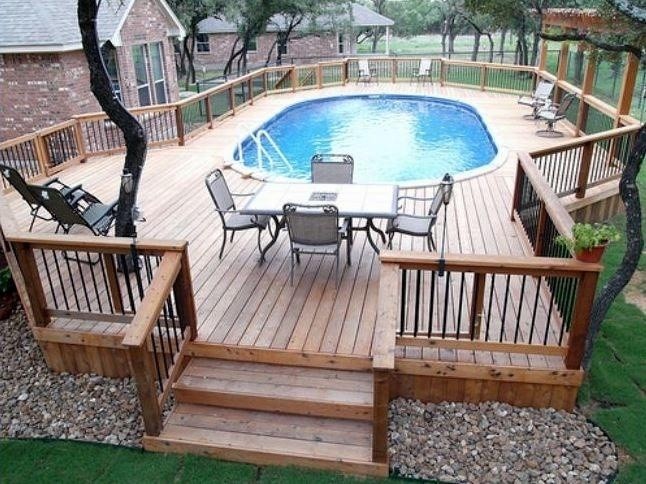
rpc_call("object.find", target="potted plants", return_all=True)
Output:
[0,267,14,320]
[549,220,622,263]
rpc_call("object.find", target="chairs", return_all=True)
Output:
[0,163,146,266]
[518,79,578,137]
[412,60,435,87]
[353,58,378,87]
[204,152,454,290]
